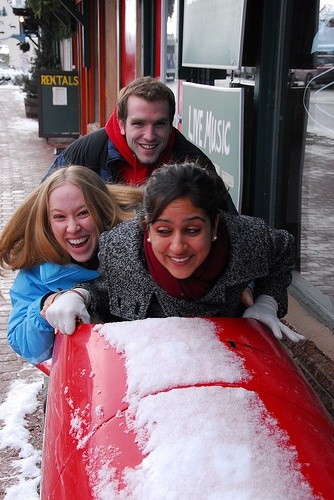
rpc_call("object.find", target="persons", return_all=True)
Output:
[0,165,143,366]
[41,74,254,309]
[43,162,307,344]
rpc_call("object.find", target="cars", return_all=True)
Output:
[290,49,334,89]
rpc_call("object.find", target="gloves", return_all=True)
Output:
[45,288,90,336]
[241,295,304,343]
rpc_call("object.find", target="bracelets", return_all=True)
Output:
[51,290,87,305]
